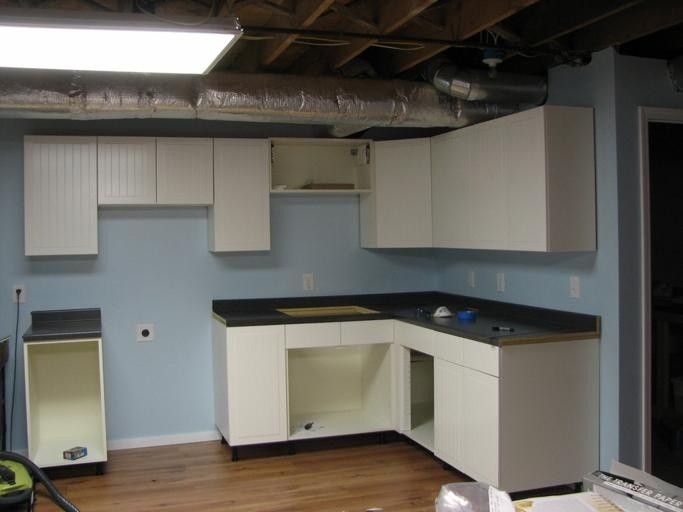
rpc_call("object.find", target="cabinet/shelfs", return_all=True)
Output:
[359,137,432,249]
[432,105,596,252]
[267,137,374,196]
[98,136,156,205]
[24,135,98,256]
[156,137,213,207]
[22,308,108,482]
[208,138,270,253]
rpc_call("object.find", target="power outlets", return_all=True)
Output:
[12,284,25,304]
[136,323,154,343]
